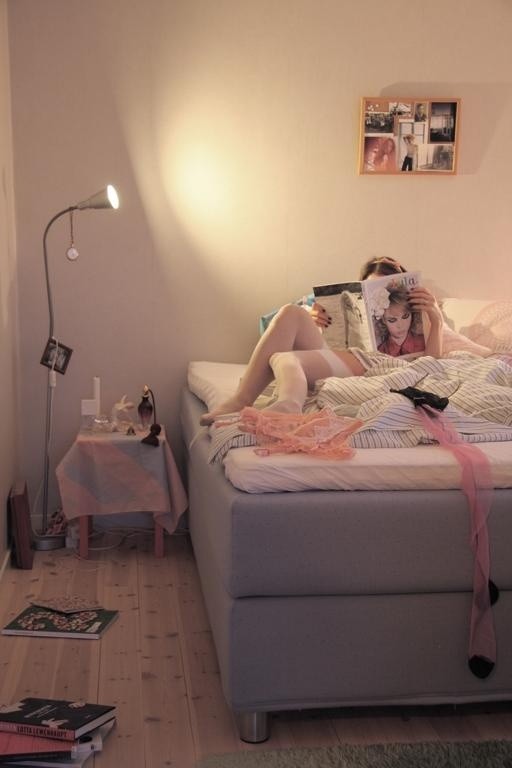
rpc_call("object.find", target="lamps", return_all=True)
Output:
[137,384,163,449]
[30,182,127,552]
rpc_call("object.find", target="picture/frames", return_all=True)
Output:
[355,94,465,177]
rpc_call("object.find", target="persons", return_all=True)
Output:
[377,139,395,171]
[198,256,444,427]
[402,134,419,172]
[415,104,426,122]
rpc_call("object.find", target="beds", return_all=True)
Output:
[175,359,512,746]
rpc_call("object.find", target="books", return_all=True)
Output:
[306,271,427,360]
[2,605,119,640]
[0,696,119,768]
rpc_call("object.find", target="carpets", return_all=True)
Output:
[196,737,512,767]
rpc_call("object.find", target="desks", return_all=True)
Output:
[54,421,190,561]
